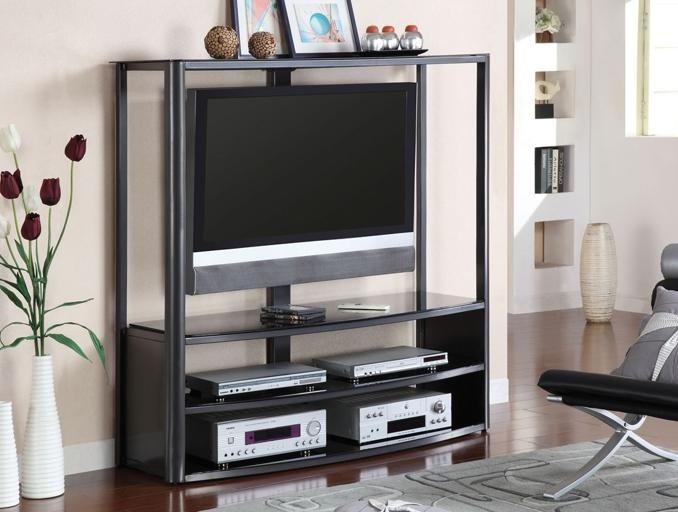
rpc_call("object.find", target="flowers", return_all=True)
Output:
[0,126,111,378]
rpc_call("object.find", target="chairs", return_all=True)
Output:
[534,242,678,498]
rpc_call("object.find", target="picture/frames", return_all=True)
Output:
[230,1,361,61]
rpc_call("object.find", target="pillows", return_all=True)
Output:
[607,285,678,387]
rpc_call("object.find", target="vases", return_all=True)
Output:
[0,355,65,509]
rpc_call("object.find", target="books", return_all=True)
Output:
[535,147,567,194]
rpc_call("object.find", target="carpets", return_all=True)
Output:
[196,437,676,511]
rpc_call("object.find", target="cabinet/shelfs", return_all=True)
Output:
[515,0,591,313]
[115,54,490,485]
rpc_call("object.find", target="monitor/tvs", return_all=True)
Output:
[185,82,418,295]
